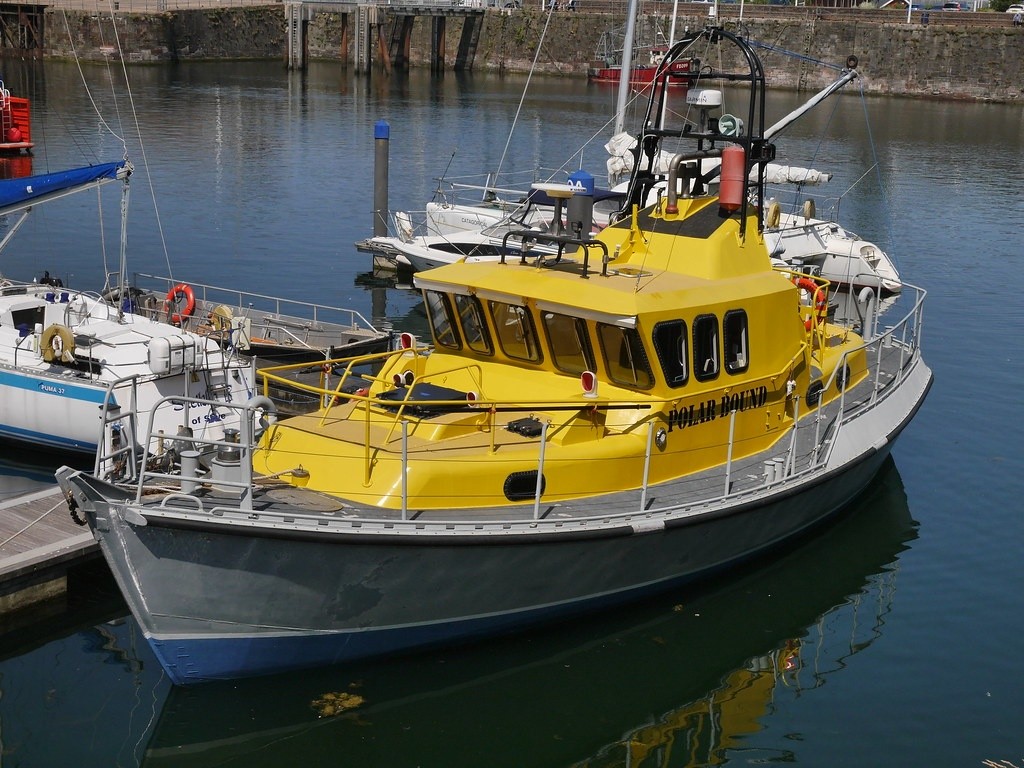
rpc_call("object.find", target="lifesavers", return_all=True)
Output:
[791,276,827,332]
[40,324,76,365]
[766,202,781,229]
[164,283,195,323]
[803,198,818,221]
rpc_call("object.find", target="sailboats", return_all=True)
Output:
[354,1,908,293]
[130,454,924,767]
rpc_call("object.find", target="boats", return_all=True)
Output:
[52,202,932,690]
[0,279,215,464]
[584,51,697,88]
[89,282,396,362]
